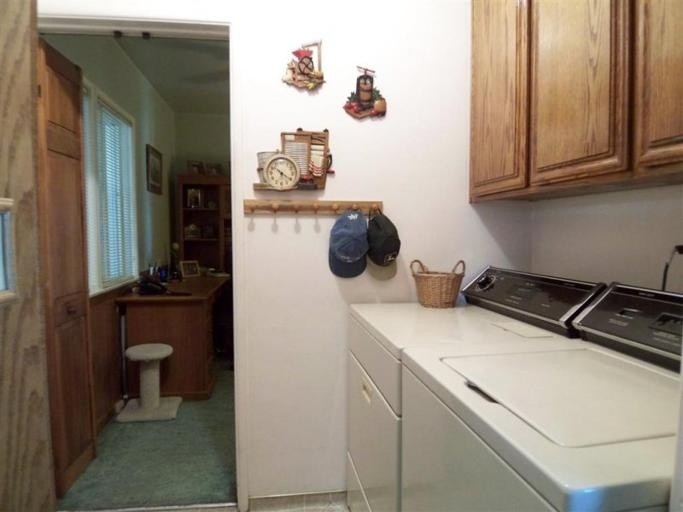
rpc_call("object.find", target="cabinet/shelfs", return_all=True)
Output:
[177,173,232,274]
[634,0,683,192]
[465,0,529,207]
[528,0,634,202]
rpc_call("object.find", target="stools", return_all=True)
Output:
[114,342,184,423]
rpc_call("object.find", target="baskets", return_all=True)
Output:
[410,259,465,309]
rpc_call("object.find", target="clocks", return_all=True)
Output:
[262,152,301,191]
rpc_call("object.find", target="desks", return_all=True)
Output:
[116,276,230,402]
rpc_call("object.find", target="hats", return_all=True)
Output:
[329,208,369,278]
[367,207,400,266]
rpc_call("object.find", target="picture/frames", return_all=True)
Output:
[146,141,164,196]
[178,258,200,277]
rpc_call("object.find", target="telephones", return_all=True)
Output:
[137,280,166,296]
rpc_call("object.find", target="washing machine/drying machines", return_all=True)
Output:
[402,280,683,512]
